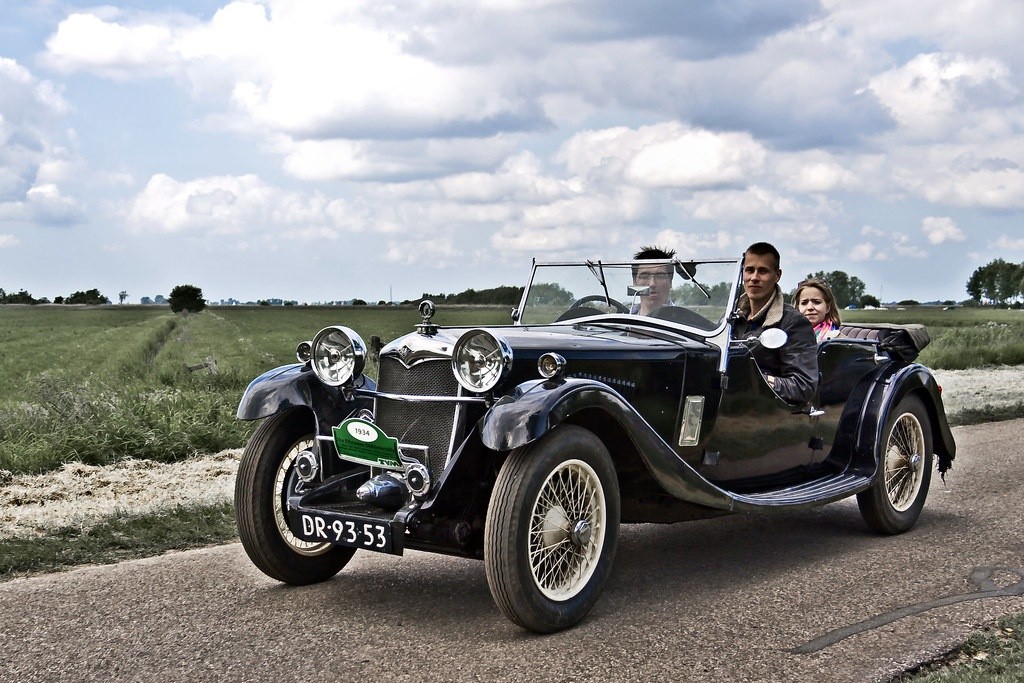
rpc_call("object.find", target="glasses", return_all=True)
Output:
[798,279,827,288]
[636,274,670,282]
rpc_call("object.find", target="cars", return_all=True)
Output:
[233,256,955,636]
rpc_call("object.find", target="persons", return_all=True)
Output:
[791,279,846,344]
[628,246,675,315]
[720,242,818,405]
